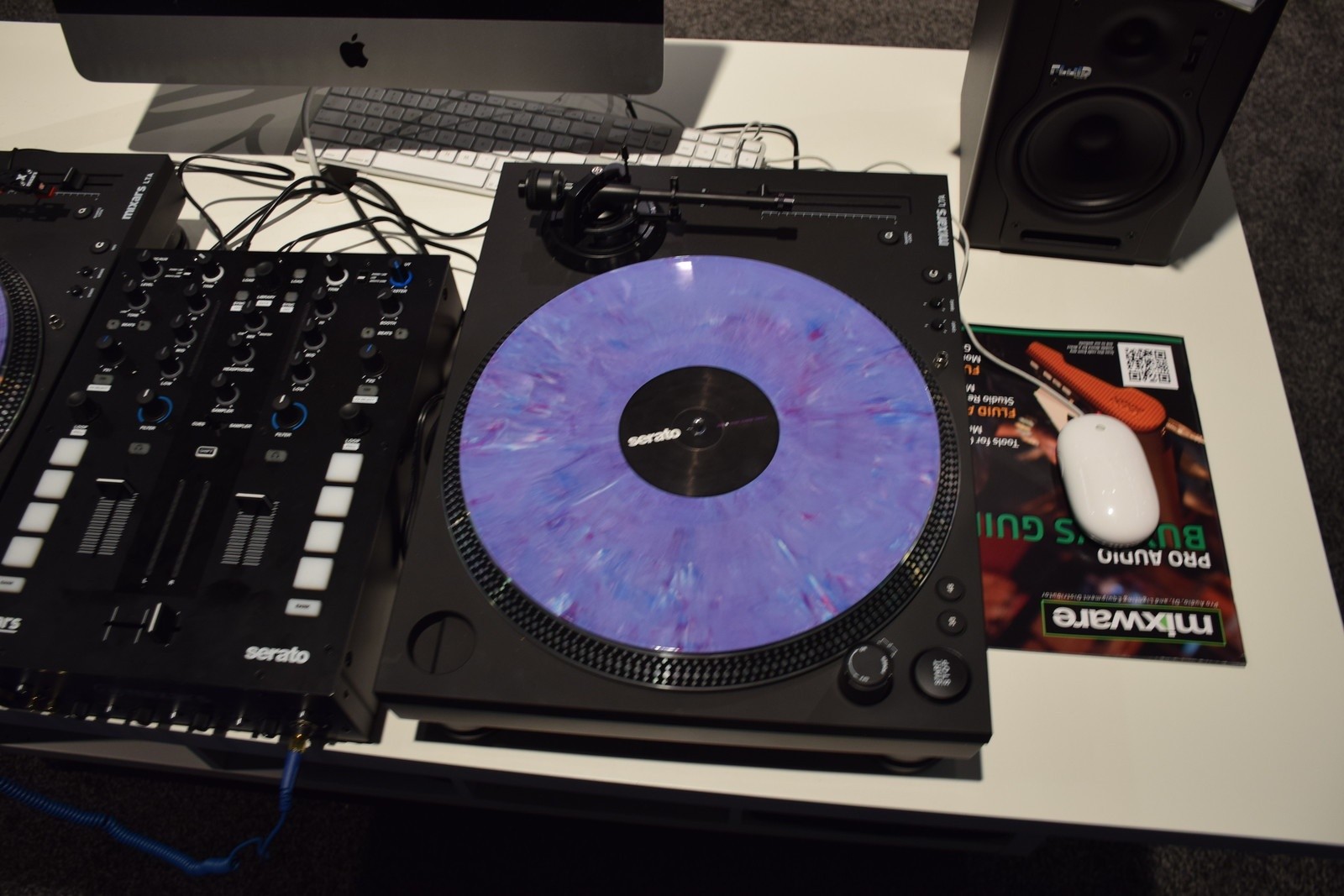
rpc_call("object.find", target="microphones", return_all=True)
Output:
[517,164,795,213]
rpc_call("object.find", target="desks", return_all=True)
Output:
[2,19,1344,895]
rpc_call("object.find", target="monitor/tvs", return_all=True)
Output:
[49,0,665,96]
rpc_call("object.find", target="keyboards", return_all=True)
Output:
[288,90,766,199]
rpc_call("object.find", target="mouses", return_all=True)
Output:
[1054,411,1162,548]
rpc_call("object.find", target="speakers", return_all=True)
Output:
[956,0,1290,266]
